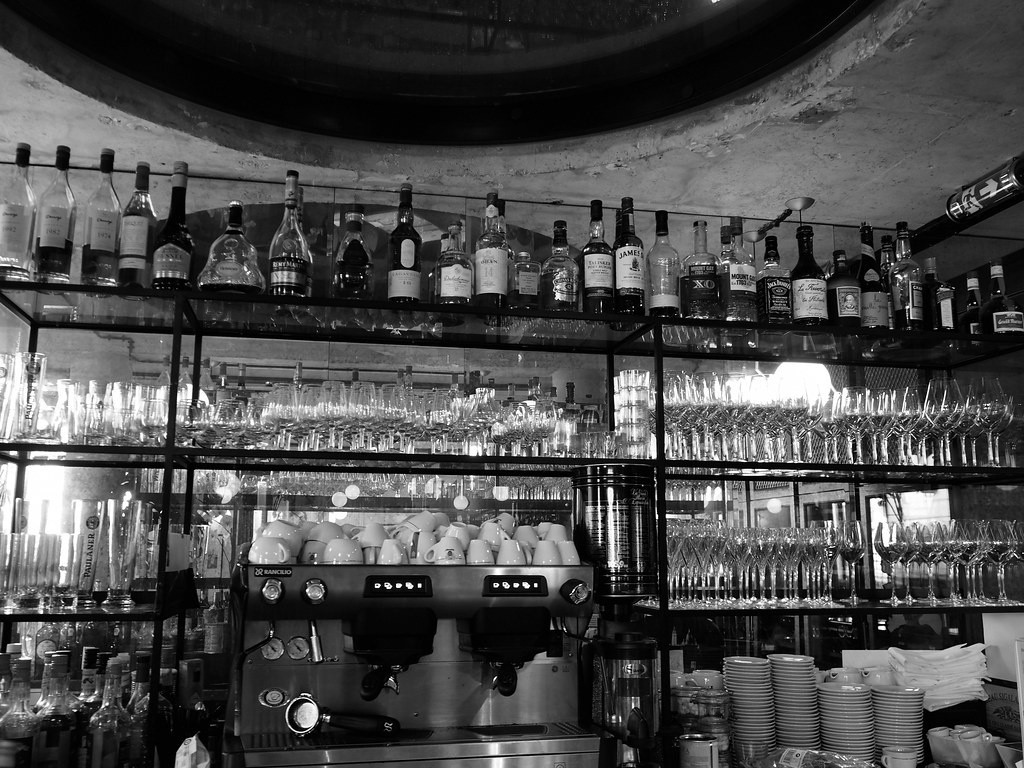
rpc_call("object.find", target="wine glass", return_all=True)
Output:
[875,519,1024,608]
[636,518,868,609]
[139,380,559,458]
[650,370,1024,468]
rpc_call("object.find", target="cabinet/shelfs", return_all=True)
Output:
[0,280,1024,768]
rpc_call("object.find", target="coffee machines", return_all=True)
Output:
[579,628,664,768]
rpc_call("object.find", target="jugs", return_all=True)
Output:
[671,688,733,768]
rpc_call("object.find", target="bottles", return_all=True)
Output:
[0,142,1023,356]
[0,644,180,768]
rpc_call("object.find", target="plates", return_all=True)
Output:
[722,654,821,767]
[815,682,926,765]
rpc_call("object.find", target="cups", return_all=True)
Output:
[927,724,1001,767]
[1,349,191,447]
[881,747,917,768]
[668,669,725,693]
[816,666,895,685]
[246,510,580,566]
[556,368,653,461]
[1,496,211,612]
